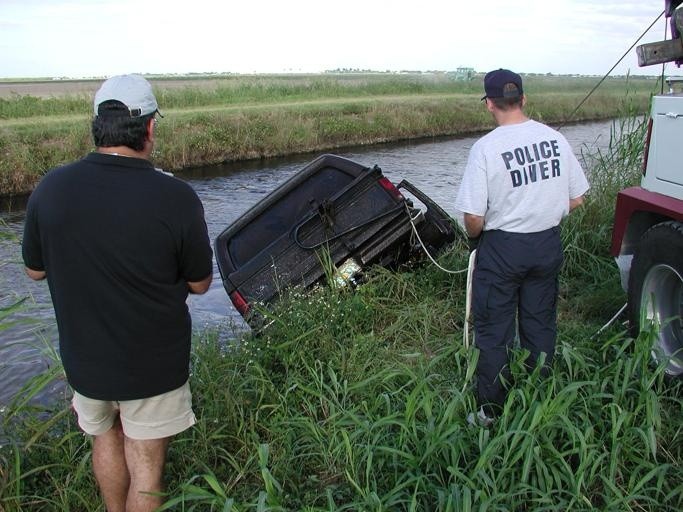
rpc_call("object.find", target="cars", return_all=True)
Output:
[212,154,468,341]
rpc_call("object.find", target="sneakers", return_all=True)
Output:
[466,406,502,429]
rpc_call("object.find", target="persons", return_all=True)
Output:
[22,74,213,512]
[454,68,590,427]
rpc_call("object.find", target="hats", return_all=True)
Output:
[93,73,165,123]
[479,68,524,103]
